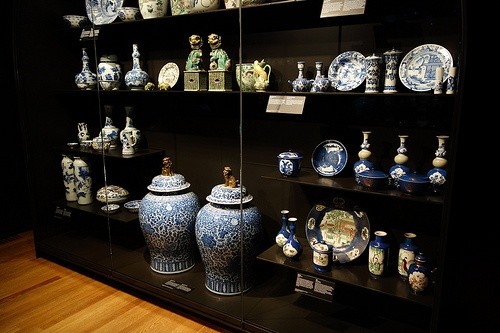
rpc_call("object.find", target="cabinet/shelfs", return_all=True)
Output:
[22,0,500,333]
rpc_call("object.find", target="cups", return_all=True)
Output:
[235,64,255,90]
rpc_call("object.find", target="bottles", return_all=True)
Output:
[62,105,262,296]
[291,61,329,93]
[276,209,334,272]
[74,44,149,90]
[354,130,450,294]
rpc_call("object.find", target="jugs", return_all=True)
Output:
[252,60,271,91]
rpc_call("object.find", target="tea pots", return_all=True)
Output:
[77,123,90,146]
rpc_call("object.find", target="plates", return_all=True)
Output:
[305,197,370,264]
[158,62,179,88]
[328,51,367,92]
[85,0,123,26]
[399,43,453,92]
[310,140,348,177]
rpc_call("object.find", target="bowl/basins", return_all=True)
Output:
[63,15,87,27]
[101,204,120,213]
[357,168,389,190]
[91,136,111,152]
[277,150,303,179]
[67,143,78,149]
[124,200,141,212]
[398,170,433,195]
[116,7,139,22]
